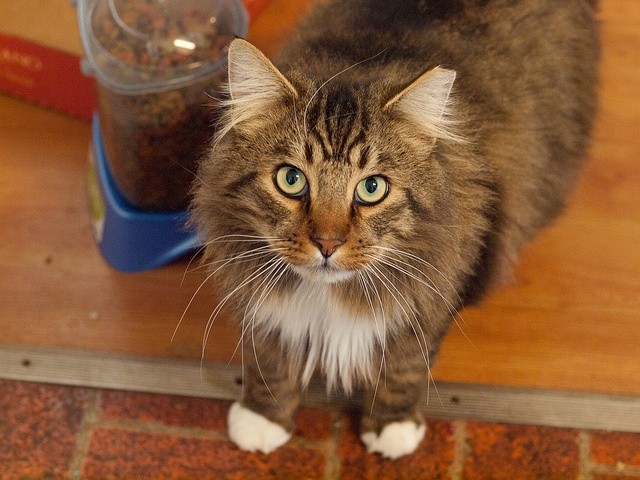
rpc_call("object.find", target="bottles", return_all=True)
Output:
[73,0,246,214]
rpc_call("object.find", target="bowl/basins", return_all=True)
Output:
[88,113,212,272]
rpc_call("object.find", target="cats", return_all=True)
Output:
[172,0,601,461]
